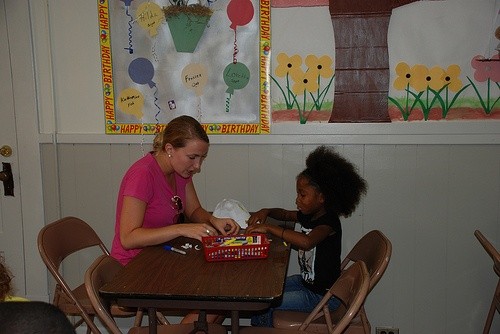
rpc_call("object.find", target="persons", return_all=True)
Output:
[245,145,369,328]
[0,255,30,302]
[109,115,240,276]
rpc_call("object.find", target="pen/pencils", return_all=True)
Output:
[163,245,187,255]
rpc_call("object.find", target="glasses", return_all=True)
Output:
[170,196,185,224]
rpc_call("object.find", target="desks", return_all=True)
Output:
[99,212,296,334]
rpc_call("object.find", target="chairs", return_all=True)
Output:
[37,216,392,334]
[474,230,500,334]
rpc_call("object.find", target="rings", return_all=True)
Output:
[206,229,210,234]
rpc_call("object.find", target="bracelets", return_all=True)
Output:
[282,229,285,240]
